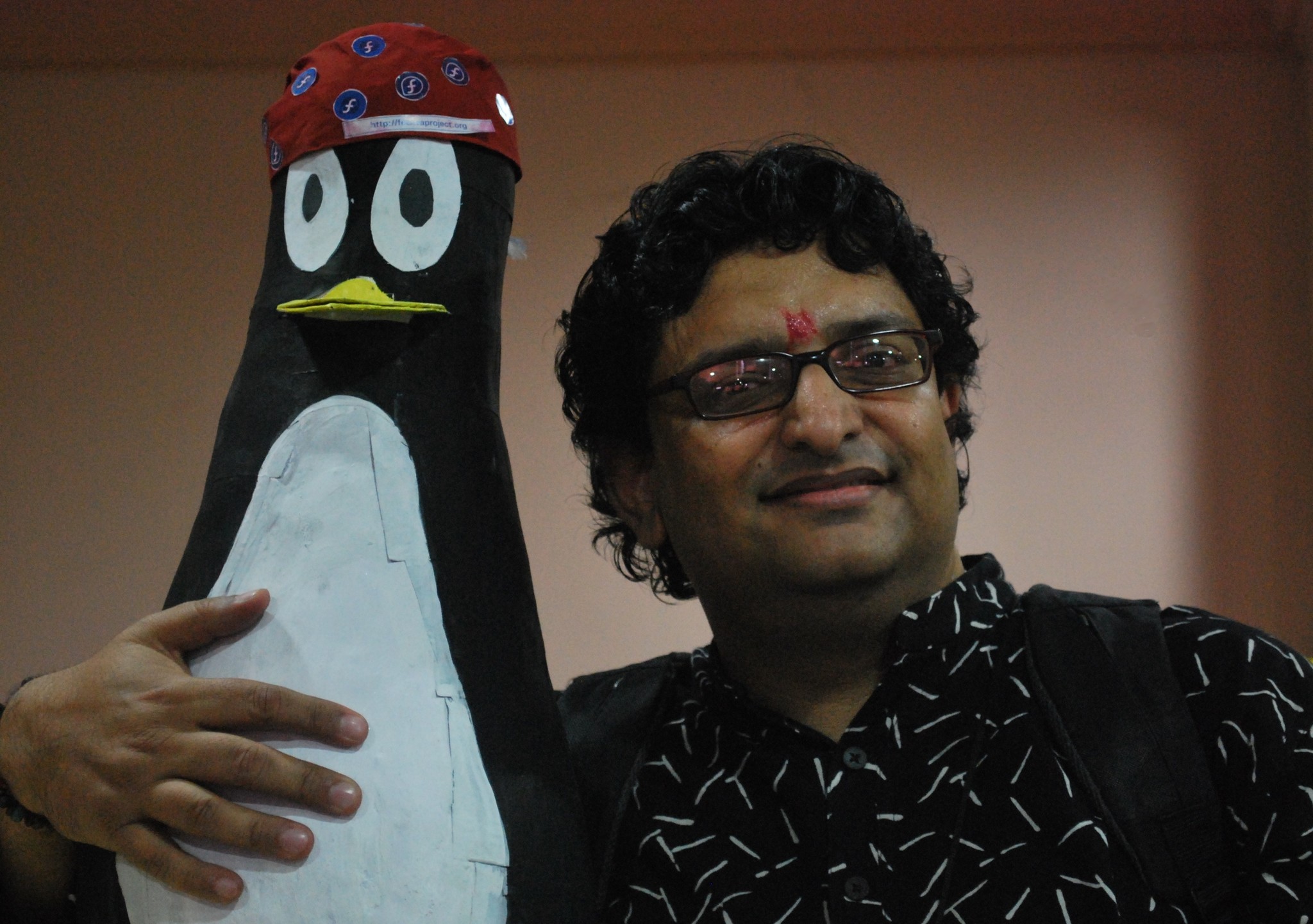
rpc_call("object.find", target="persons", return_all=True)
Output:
[0,132,1313,923]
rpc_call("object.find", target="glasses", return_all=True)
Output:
[651,328,944,426]
